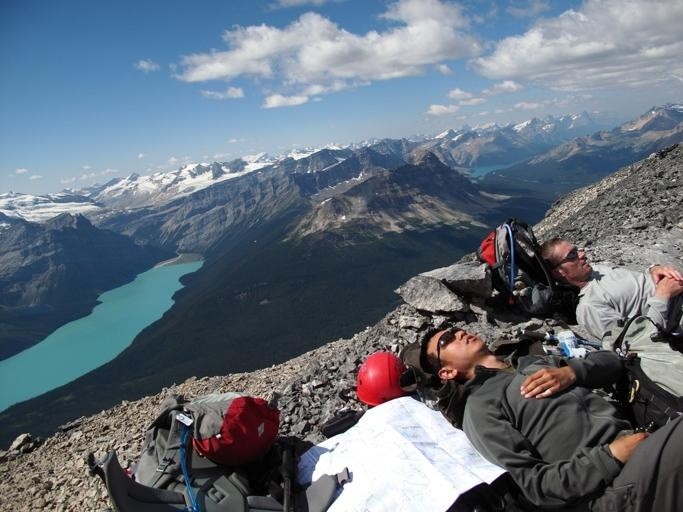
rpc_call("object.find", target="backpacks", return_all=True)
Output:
[87,391,352,512]
[474,216,561,320]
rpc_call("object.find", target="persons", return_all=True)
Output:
[539,237,683,341]
[420,322,683,512]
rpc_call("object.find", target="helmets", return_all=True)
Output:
[356,350,409,407]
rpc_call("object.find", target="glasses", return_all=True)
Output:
[553,246,579,270]
[436,325,462,369]
